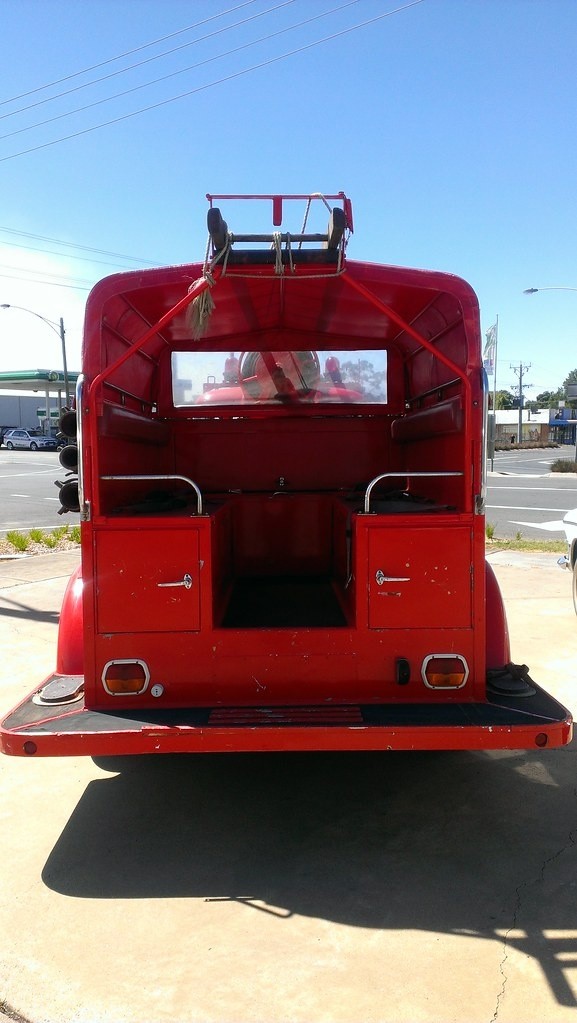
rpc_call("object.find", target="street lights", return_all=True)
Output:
[0,302,70,408]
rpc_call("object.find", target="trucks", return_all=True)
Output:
[0,187,577,759]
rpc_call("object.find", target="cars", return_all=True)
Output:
[0,425,59,451]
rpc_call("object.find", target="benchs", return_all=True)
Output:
[97,498,236,630]
[331,493,471,629]
